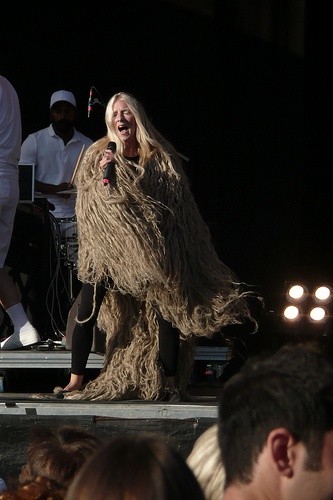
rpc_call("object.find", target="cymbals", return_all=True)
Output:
[56,189,77,194]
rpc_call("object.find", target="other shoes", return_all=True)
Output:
[54,387,81,399]
[161,391,183,402]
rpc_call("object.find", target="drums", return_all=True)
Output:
[0,204,61,341]
[56,218,77,270]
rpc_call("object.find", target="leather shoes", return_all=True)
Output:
[0,328,41,350]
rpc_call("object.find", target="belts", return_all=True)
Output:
[55,218,77,223]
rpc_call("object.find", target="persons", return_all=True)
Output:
[53,92,184,401]
[0,75,42,350]
[18,90,94,340]
[0,341,333,500]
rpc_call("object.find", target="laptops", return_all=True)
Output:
[18,162,35,204]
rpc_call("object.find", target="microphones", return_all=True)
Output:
[88,88,93,117]
[103,142,116,186]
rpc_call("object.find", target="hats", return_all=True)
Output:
[50,90,76,109]
